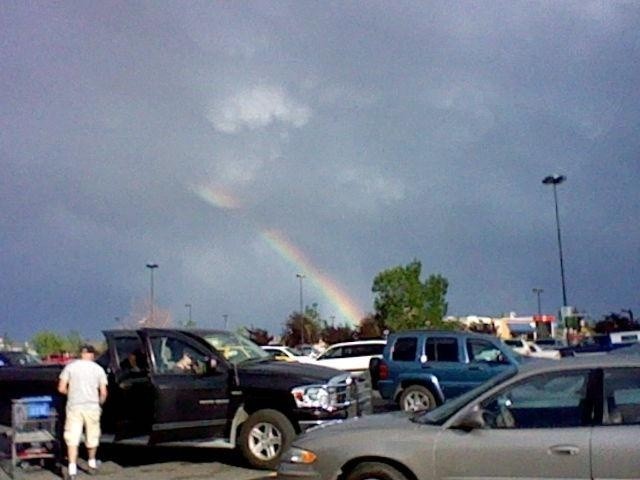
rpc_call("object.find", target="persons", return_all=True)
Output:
[57,344,110,480]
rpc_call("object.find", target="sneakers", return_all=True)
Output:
[66,460,102,479]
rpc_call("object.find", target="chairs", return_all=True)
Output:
[165,344,180,369]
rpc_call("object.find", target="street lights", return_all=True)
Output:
[184,304,192,322]
[532,174,575,316]
[221,314,229,330]
[145,263,158,321]
[296,273,308,344]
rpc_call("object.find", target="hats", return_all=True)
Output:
[81,343,98,354]
